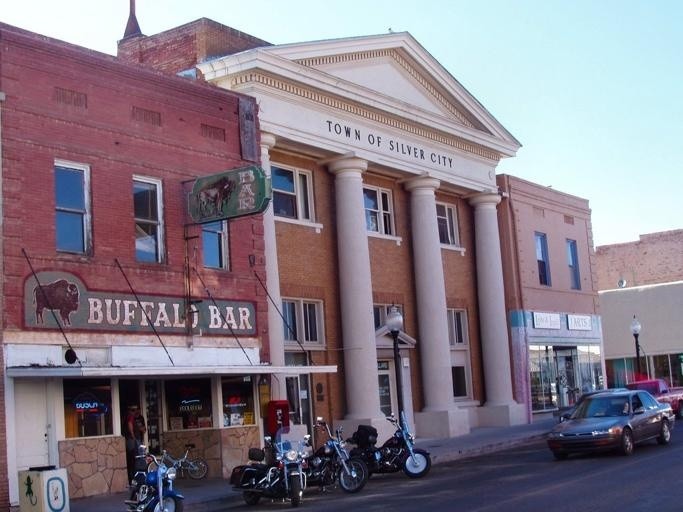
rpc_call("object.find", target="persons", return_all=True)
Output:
[123,404,139,451]
[134,407,146,441]
[622,402,629,414]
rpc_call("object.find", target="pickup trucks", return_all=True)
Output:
[623,377,682,423]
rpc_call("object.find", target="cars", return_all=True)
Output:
[544,388,675,465]
[559,385,633,425]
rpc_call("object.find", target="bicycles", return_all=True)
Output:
[144,442,211,483]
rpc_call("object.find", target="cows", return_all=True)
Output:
[32,278,79,328]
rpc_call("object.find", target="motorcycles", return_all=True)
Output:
[122,443,186,512]
[228,423,315,509]
[264,414,370,497]
[344,405,435,483]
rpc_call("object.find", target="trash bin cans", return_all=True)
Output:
[18,465,70,512]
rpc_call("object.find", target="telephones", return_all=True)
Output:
[277,409,282,424]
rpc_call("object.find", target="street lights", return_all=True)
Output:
[629,314,643,382]
[385,298,404,428]
